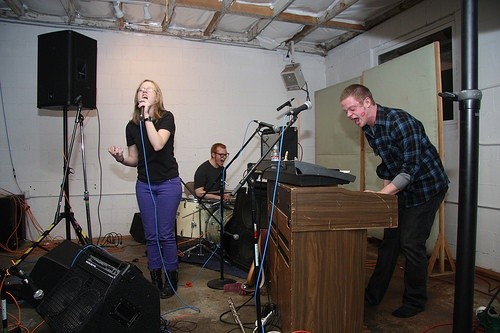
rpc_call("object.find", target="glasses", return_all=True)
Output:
[138,87,158,94]
[214,152,230,158]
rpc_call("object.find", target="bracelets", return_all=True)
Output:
[118,160,124,162]
[144,116,153,121]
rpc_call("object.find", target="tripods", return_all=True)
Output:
[7,109,91,270]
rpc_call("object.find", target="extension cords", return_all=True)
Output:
[102,243,125,248]
[255,310,275,327]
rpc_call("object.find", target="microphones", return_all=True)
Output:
[285,101,312,117]
[254,120,280,133]
[276,98,295,111]
[218,230,240,240]
[140,106,144,121]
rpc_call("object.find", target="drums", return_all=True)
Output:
[176,196,240,251]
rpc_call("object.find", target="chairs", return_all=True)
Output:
[185,181,210,238]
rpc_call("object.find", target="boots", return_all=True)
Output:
[150,269,163,291]
[160,270,178,298]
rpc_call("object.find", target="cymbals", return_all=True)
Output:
[199,189,233,194]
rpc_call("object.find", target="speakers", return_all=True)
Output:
[226,186,268,274]
[129,213,146,244]
[37,30,97,109]
[35,247,162,333]
[23,240,85,306]
[281,63,306,91]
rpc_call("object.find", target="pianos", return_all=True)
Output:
[252,158,398,333]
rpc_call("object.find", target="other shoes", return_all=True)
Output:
[392,306,425,317]
[364,288,378,308]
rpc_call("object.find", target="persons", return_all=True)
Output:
[341,84,451,317]
[109,80,183,299]
[193,143,231,200]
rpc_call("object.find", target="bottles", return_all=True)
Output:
[270,144,280,169]
